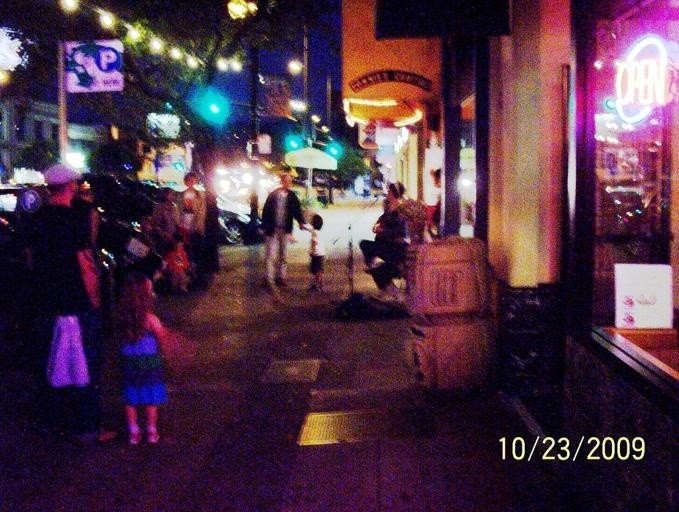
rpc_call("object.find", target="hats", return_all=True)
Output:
[44,164,82,185]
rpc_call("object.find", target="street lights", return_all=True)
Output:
[227,0,329,177]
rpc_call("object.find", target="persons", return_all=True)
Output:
[359,169,441,299]
[261,173,307,288]
[23,171,208,443]
[303,214,324,292]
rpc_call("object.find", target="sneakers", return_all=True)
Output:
[147,431,159,445]
[280,277,289,286]
[82,431,116,445]
[128,429,143,444]
[308,284,321,292]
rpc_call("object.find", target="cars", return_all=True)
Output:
[132,180,263,245]
[293,178,329,206]
